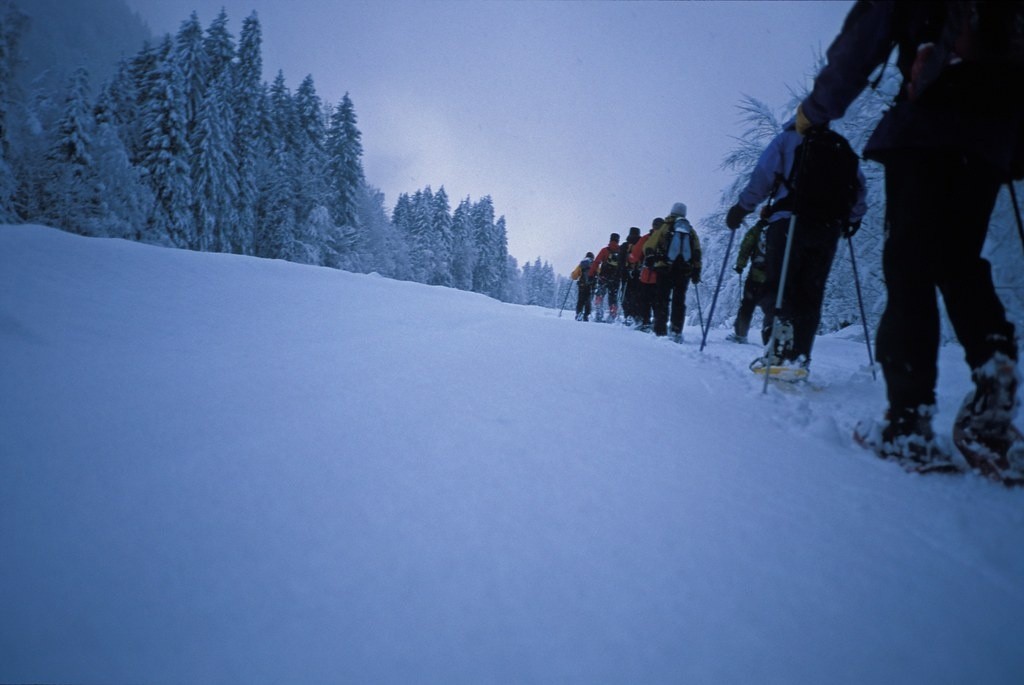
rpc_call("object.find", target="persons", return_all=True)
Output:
[642,203,701,337]
[726,116,867,380]
[587,233,620,322]
[786,0,1024,484]
[618,227,641,326]
[726,206,771,347]
[627,218,664,333]
[571,252,599,321]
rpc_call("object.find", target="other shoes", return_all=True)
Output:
[781,352,812,370]
[750,353,785,369]
[854,406,937,460]
[668,331,684,344]
[575,312,616,324]
[726,333,747,344]
[968,354,1022,444]
[622,316,656,333]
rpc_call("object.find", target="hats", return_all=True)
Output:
[671,202,686,216]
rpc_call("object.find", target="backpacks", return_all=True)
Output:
[600,247,622,282]
[793,128,857,223]
[907,0,1024,101]
[660,217,697,265]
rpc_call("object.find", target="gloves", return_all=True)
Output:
[796,103,813,134]
[842,220,861,238]
[736,266,744,274]
[727,203,750,230]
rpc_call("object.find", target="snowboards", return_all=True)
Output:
[756,365,810,382]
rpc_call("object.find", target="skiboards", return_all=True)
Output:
[853,419,1024,487]
